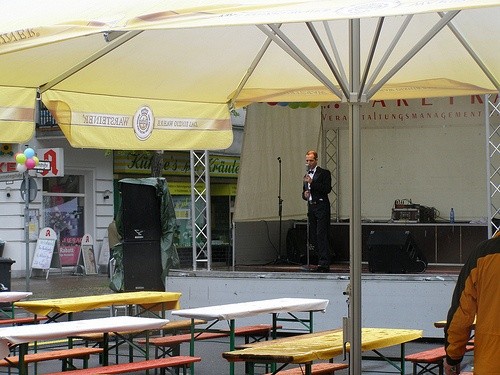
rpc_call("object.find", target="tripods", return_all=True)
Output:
[266,162,302,266]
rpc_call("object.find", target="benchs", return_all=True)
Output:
[0,318,475,375]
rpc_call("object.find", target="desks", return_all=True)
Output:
[222,327,423,375]
[0,315,171,375]
[13,292,182,336]
[171,298,330,375]
[0,292,33,318]
[434,314,477,373]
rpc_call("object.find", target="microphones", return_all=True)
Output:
[277,157,281,163]
[305,164,309,172]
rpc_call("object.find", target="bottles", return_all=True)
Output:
[449,208,454,223]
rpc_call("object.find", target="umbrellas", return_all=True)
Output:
[0,3,500,375]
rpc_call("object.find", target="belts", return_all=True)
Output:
[308,200,321,204]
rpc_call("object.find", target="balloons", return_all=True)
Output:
[14,147,39,174]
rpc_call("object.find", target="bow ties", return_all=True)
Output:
[308,170,314,174]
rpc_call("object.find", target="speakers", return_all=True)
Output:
[366,230,430,273]
[121,182,165,293]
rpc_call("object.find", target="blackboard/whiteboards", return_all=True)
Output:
[31,238,62,269]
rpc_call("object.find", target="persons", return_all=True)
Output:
[443,230,500,375]
[302,150,332,272]
